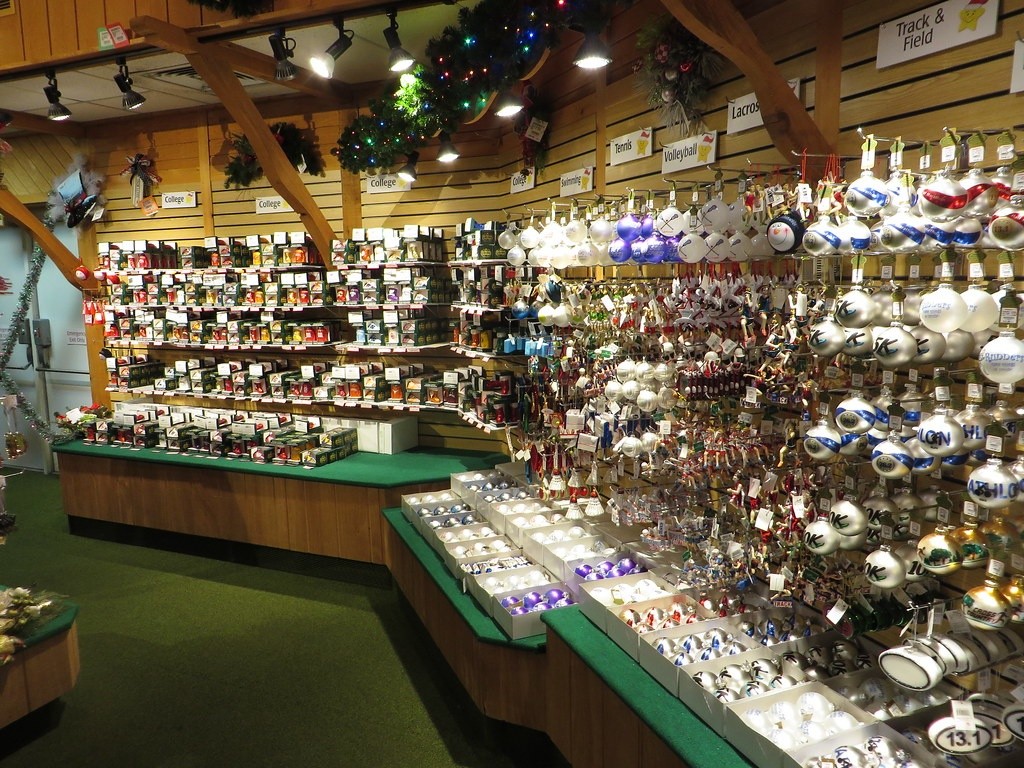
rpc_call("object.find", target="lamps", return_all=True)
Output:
[309,20,355,79]
[397,150,420,182]
[434,132,461,163]
[113,58,147,111]
[493,92,525,118]
[567,24,613,76]
[269,29,298,82]
[382,12,416,72]
[41,71,72,121]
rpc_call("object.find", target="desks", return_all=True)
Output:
[381,507,756,768]
[52,436,508,565]
[0,585,81,729]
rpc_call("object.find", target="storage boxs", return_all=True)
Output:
[564,550,646,596]
[495,456,964,768]
[451,470,500,496]
[543,535,611,582]
[523,520,603,566]
[412,499,576,640]
[488,498,547,535]
[95,229,507,467]
[462,477,517,509]
[606,594,719,661]
[638,618,761,696]
[401,489,461,522]
[578,572,680,633]
[475,487,529,520]
[504,508,568,549]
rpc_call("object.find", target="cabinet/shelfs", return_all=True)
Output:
[94,259,519,462]
